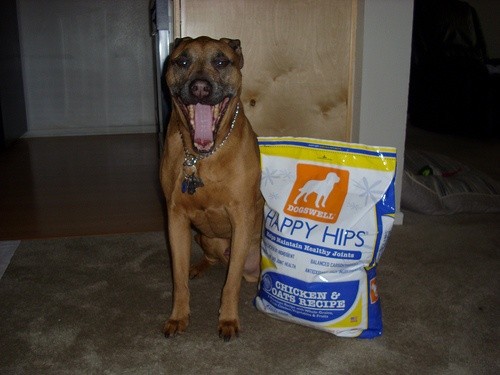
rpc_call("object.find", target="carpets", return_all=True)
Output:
[0,206,500,375]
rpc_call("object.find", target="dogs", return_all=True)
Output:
[157,35,266,343]
